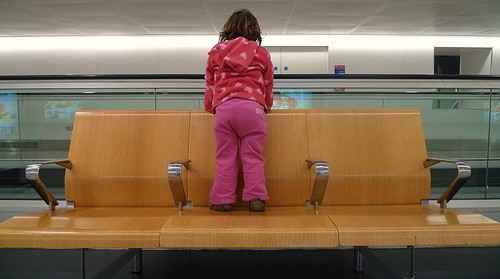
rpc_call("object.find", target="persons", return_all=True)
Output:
[205,9,274,212]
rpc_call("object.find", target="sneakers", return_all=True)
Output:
[208,200,232,211]
[249,198,266,212]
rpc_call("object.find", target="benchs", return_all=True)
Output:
[0,109,500,279]
